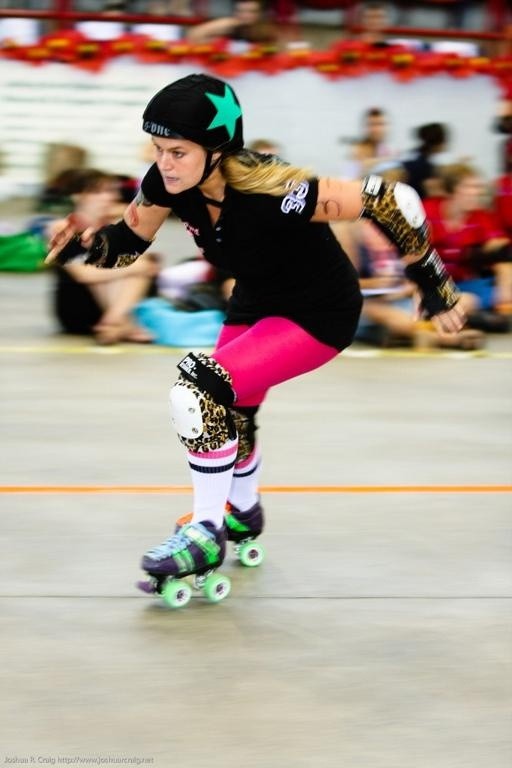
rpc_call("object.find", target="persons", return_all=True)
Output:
[41,107,512,354]
[48,74,468,575]
[338,2,396,49]
[184,0,291,49]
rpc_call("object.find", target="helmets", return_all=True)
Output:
[142,74,244,155]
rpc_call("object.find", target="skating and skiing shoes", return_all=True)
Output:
[136,496,265,607]
